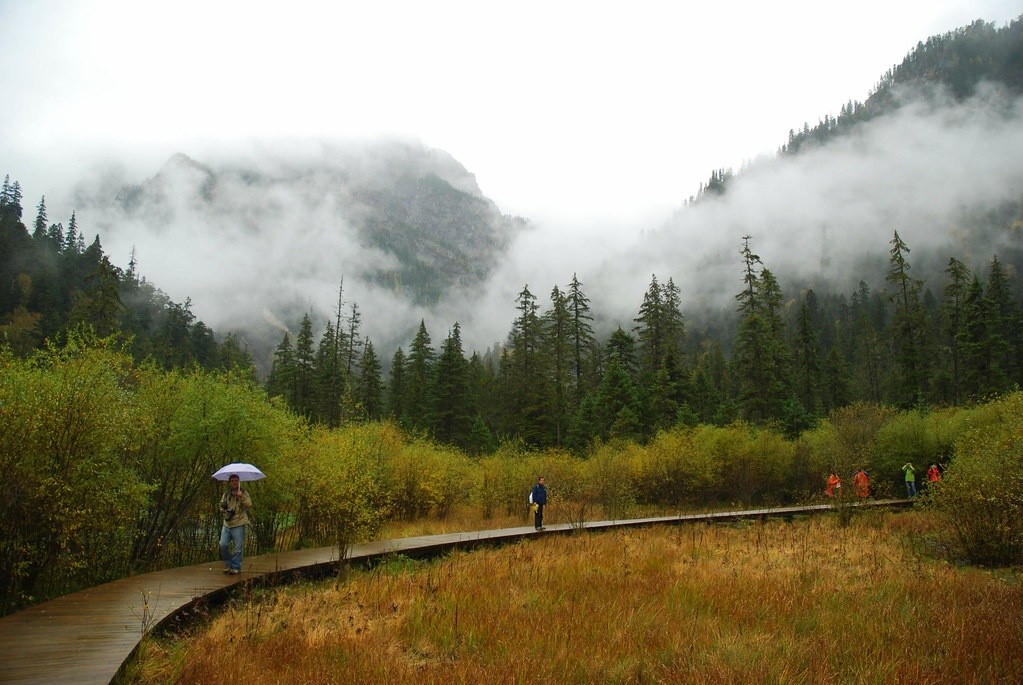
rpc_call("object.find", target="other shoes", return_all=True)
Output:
[223,567,231,573]
[537,527,543,530]
[542,526,546,529]
[229,569,241,574]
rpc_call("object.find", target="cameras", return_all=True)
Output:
[225,509,235,522]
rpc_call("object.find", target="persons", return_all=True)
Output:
[532,477,547,531]
[824,470,842,499]
[902,463,916,499]
[927,463,939,483]
[853,468,868,503]
[219,475,253,574]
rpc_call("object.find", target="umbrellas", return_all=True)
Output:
[211,463,266,493]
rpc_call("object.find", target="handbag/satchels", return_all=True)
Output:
[532,503,539,513]
[529,492,533,504]
